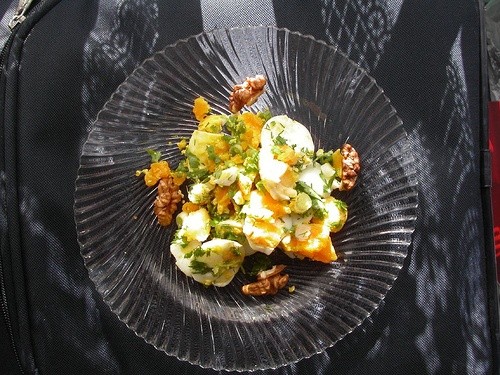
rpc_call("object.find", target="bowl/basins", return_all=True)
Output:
[72,24,419,375]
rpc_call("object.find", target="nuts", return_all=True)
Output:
[229,75,267,115]
[242,265,289,297]
[340,144,360,192]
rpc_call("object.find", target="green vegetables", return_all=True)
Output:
[146,110,350,287]
